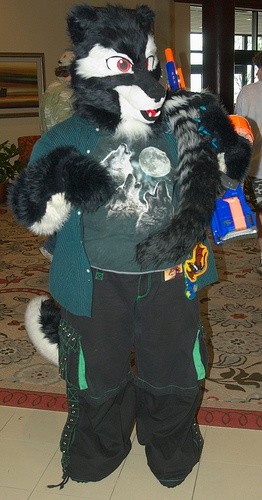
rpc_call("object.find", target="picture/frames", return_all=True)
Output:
[0,51,47,119]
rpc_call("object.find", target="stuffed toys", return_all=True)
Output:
[9,1,255,489]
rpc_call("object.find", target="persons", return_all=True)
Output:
[39,51,76,263]
[233,50,262,270]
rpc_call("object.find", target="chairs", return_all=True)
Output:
[18,134,39,165]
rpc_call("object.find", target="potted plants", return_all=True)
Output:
[0,141,26,203]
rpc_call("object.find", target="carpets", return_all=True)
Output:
[1,199,262,430]
